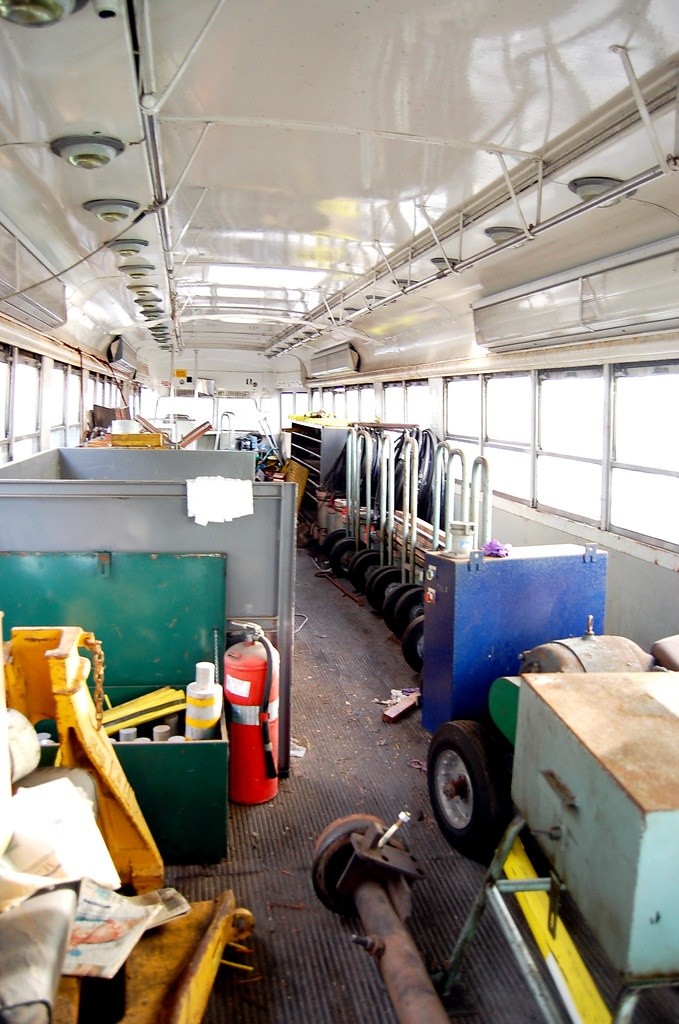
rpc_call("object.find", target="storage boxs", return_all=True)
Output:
[0,553,230,867]
[512,671,679,981]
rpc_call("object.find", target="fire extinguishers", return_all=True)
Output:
[222,620,283,805]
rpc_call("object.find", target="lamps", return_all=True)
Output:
[0,0,89,27]
[1,223,67,332]
[484,227,526,249]
[104,239,149,257]
[126,284,171,350]
[50,136,126,169]
[310,342,359,378]
[107,334,138,373]
[117,265,156,280]
[83,199,140,222]
[431,258,472,271]
[472,235,679,353]
[568,177,636,207]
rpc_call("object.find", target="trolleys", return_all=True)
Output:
[317,426,493,673]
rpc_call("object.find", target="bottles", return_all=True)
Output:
[103,713,184,742]
[185,661,222,739]
